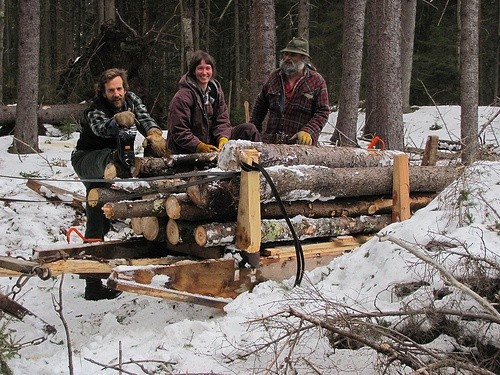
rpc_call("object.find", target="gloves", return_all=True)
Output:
[217,136,228,150]
[288,130,313,145]
[142,126,167,157]
[196,142,220,152]
[113,111,135,128]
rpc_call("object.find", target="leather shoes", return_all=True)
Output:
[117,129,135,167]
[84,281,123,301]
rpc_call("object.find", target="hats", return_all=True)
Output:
[280,37,313,60]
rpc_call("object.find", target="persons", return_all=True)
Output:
[247,36,331,150]
[71,64,166,301]
[164,50,262,159]
